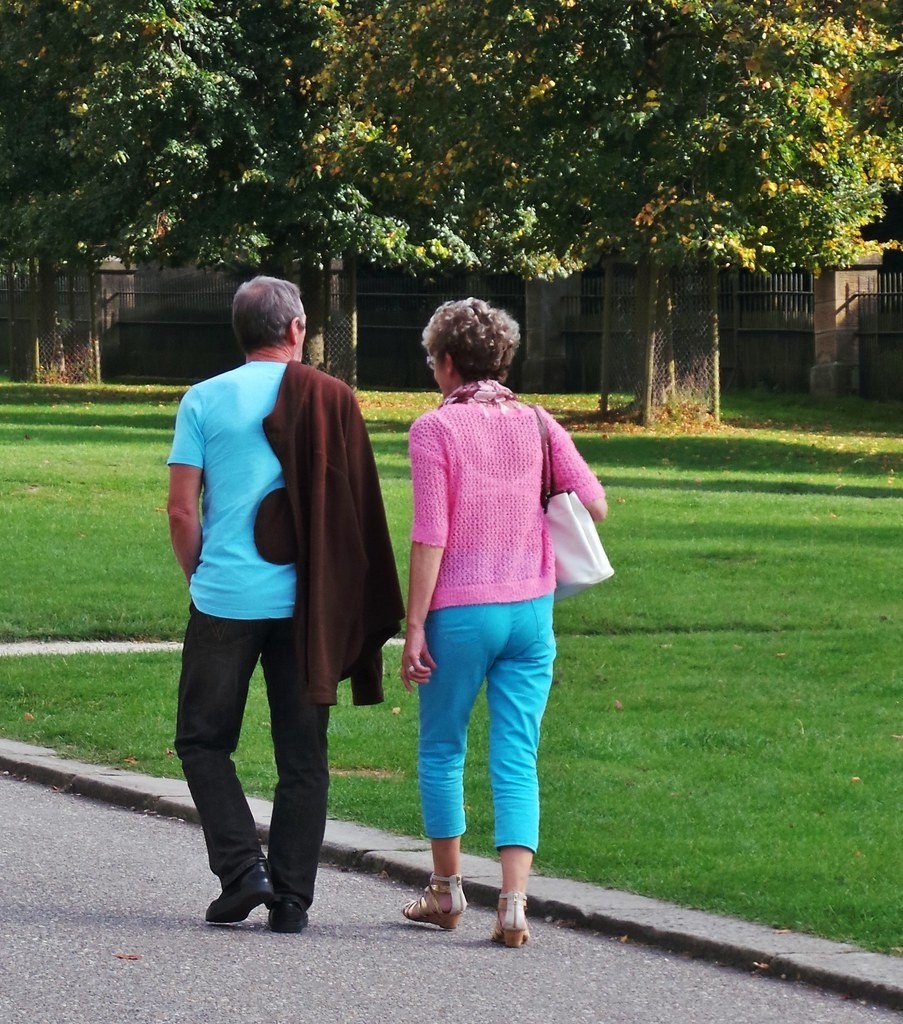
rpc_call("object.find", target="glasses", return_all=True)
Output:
[426,356,435,370]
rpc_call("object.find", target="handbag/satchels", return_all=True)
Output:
[527,403,614,600]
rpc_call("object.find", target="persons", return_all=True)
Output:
[399,295,610,947]
[166,277,372,930]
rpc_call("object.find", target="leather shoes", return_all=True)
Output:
[269,901,308,932]
[206,858,275,923]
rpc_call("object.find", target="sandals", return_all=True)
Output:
[401,873,467,928]
[491,891,529,947]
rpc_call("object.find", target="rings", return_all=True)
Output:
[408,665,415,673]
